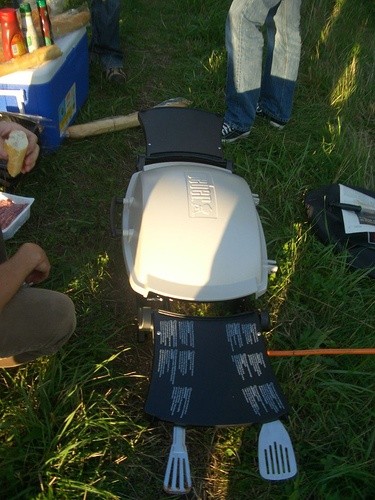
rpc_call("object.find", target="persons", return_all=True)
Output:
[218,0,300,143]
[0,120,77,369]
[85,0,127,88]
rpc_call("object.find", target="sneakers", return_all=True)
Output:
[88,44,104,63]
[221,120,251,142]
[104,66,128,86]
[255,103,288,130]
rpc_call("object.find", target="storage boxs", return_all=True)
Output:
[0,27,91,150]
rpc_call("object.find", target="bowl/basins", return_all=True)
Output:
[0,191,35,240]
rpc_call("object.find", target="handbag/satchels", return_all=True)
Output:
[305,184,375,279]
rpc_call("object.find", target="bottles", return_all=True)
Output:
[18,3,40,54]
[35,0,53,47]
[0,7,29,62]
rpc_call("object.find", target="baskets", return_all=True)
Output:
[0,192,35,241]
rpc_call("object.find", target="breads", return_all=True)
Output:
[0,45,63,77]
[14,0,91,39]
[5,130,28,177]
